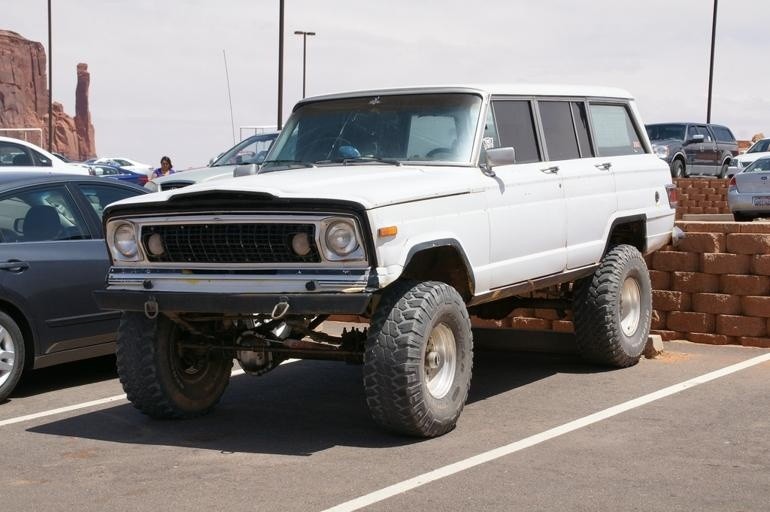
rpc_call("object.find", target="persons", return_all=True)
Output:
[150,156,175,182]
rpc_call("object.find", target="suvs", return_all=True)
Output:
[91,82,679,441]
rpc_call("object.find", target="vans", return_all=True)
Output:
[644,123,739,180]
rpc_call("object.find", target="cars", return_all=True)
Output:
[726,136,770,179]
[726,154,770,222]
[143,129,283,193]
[0,173,155,403]
[0,136,155,187]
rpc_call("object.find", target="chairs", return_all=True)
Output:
[21,203,63,239]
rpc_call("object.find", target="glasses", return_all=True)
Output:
[162,162,169,166]
